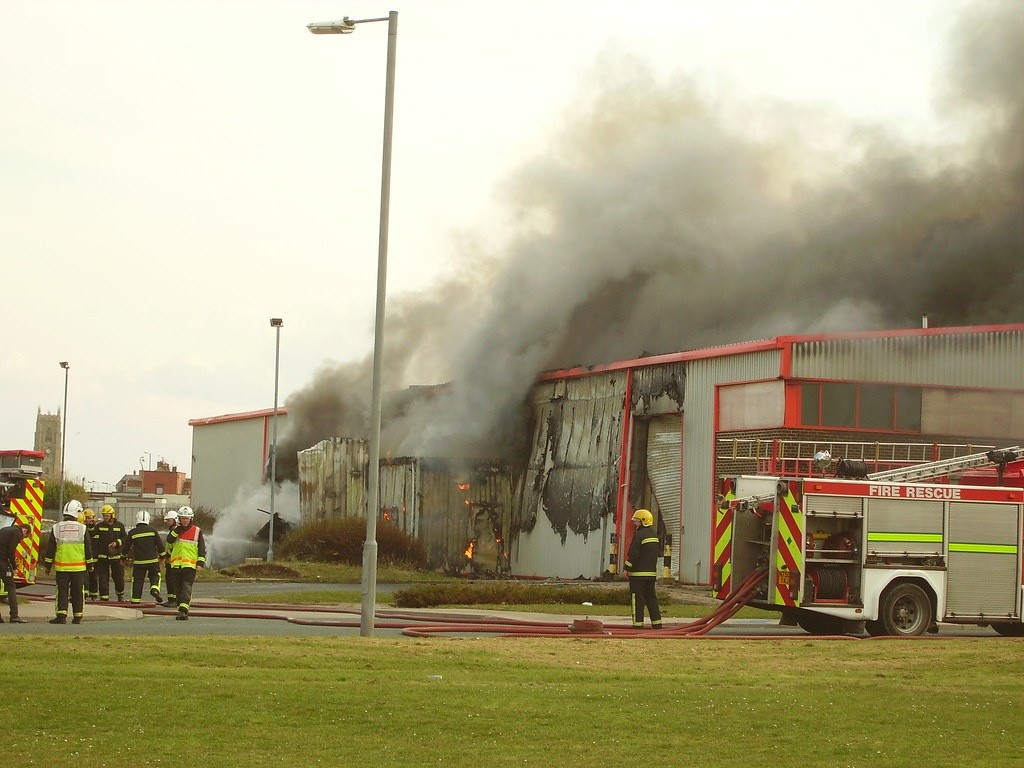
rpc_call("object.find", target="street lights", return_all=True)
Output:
[307,11,399,635]
[102,482,110,492]
[144,451,151,471]
[58,361,69,522]
[88,480,99,491]
[265,318,284,561]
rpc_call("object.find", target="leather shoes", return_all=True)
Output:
[0,618,7,623]
[10,617,28,623]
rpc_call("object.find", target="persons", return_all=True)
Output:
[162,511,180,608]
[44,499,95,624]
[167,506,207,621]
[77,505,128,603]
[121,510,166,604]
[622,509,663,630]
[0,523,31,624]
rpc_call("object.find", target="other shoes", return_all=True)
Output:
[118,597,125,602]
[151,591,164,602]
[652,624,663,629]
[50,613,67,624]
[89,595,98,601]
[72,616,82,624]
[179,611,189,620]
[168,597,177,604]
[100,597,109,601]
[130,601,140,604]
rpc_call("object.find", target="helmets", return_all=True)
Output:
[177,506,194,521]
[631,510,653,528]
[100,505,116,519]
[62,499,83,518]
[164,511,179,523]
[136,510,152,526]
[83,509,96,522]
[77,512,85,523]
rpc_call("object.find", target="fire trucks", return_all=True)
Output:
[708,436,1024,637]
[0,449,45,599]
